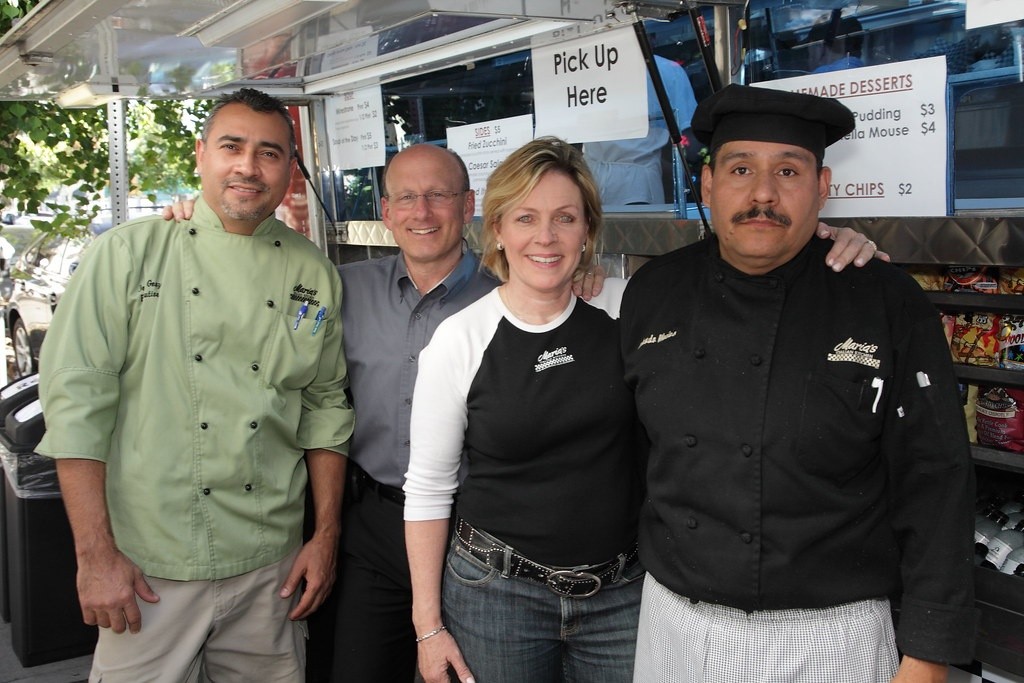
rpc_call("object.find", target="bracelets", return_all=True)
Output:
[415,625,449,643]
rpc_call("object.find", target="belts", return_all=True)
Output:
[454,514,639,599]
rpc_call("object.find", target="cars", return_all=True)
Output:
[29,178,175,236]
[0,222,112,377]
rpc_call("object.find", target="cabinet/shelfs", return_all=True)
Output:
[889,262,1024,679]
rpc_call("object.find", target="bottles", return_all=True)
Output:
[974,492,1024,577]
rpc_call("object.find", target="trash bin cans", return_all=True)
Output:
[0,396,100,667]
[0,373,40,625]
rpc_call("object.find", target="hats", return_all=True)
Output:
[691,83,856,153]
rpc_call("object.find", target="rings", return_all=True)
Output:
[866,240,879,253]
[584,271,593,278]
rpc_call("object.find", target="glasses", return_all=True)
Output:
[385,191,467,205]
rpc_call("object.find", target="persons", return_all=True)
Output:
[33,88,357,683]
[411,136,890,683]
[620,84,984,683]
[165,142,608,683]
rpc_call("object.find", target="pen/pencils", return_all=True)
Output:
[856,378,868,410]
[312,307,326,334]
[871,376,884,413]
[294,301,308,330]
